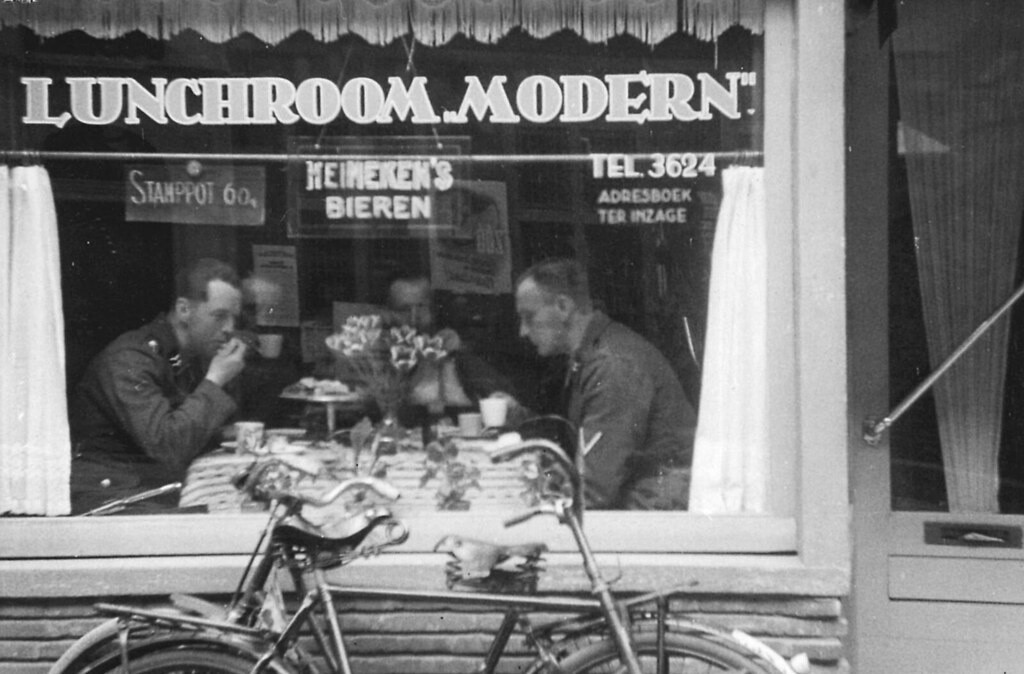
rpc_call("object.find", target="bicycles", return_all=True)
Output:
[48,416,813,674]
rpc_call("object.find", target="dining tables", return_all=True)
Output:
[179,422,538,514]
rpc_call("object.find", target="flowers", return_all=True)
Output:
[323,314,462,419]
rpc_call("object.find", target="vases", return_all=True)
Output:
[376,413,402,458]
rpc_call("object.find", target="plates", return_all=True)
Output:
[220,441,247,452]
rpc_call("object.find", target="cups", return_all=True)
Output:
[478,395,509,427]
[457,413,486,439]
[232,420,265,456]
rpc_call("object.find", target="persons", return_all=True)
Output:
[513,258,696,512]
[70,265,303,515]
[358,277,531,433]
